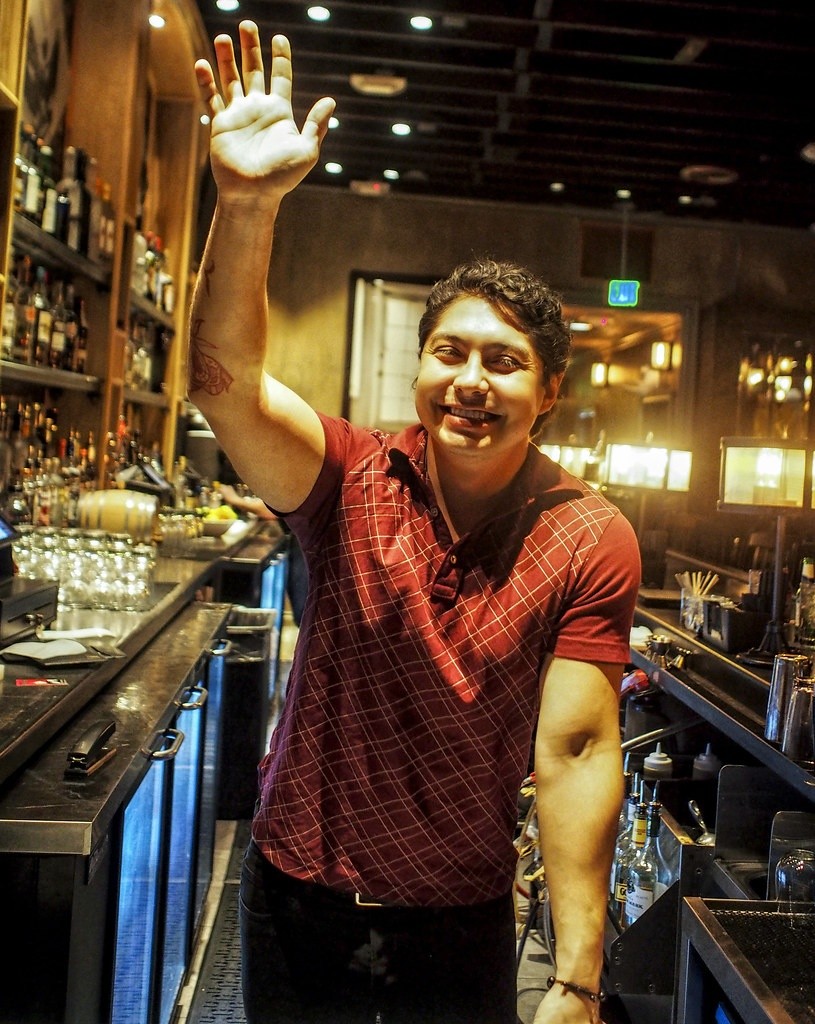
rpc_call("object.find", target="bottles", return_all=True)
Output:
[643,742,672,779]
[0,122,175,525]
[608,792,665,931]
[693,742,720,781]
[796,556,815,630]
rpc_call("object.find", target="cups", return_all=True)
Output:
[775,849,815,931]
[12,527,156,610]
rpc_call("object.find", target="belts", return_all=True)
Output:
[245,840,398,910]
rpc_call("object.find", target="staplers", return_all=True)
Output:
[64,720,117,776]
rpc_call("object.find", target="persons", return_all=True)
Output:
[189,20,641,1024]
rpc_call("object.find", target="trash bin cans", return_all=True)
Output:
[217,607,277,822]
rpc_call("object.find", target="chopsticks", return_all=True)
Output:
[674,570,720,596]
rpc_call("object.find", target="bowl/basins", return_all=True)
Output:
[203,520,233,535]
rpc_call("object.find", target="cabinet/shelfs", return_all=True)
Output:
[0,0,228,553]
[0,530,289,1024]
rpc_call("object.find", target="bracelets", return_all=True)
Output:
[546,975,605,1003]
